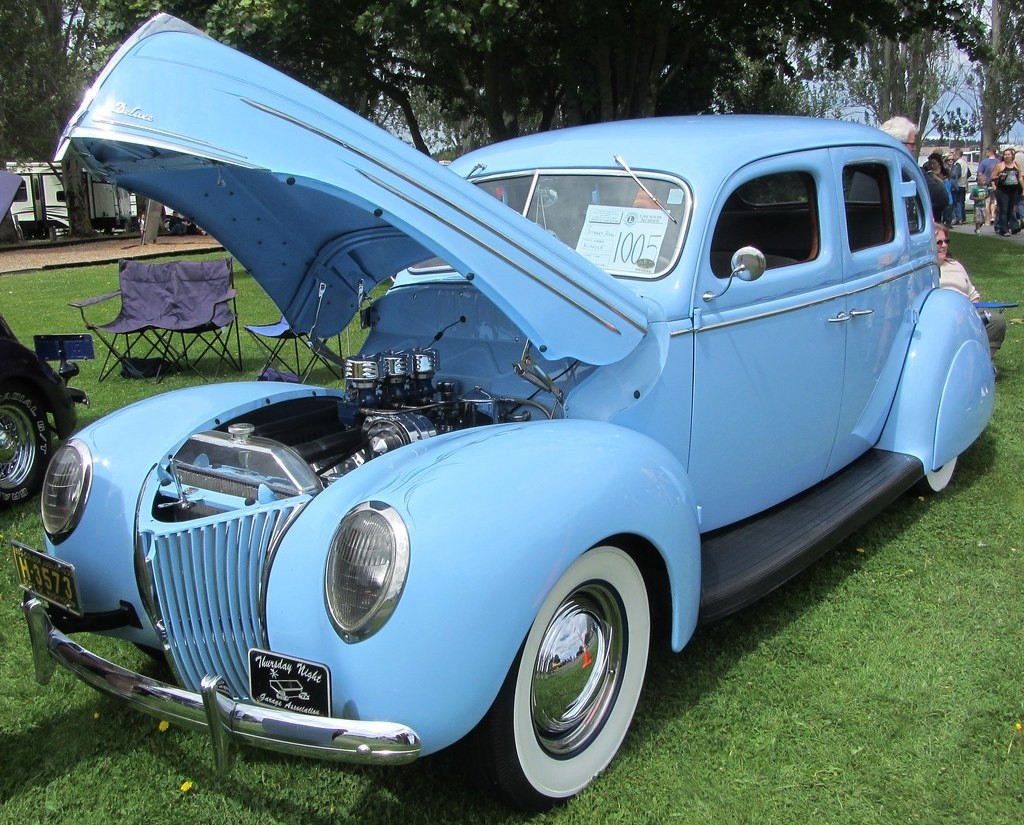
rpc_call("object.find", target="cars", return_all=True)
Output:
[0,313,95,517]
[942,150,980,182]
[7,11,996,814]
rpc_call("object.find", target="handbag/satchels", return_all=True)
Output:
[967,168,971,178]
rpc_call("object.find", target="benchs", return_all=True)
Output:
[723,206,885,261]
[67,256,243,384]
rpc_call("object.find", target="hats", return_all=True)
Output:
[1014,144,1024,153]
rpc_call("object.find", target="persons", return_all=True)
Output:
[933,222,1006,378]
[138,214,203,236]
[878,116,1024,237]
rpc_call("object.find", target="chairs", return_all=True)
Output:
[243,314,345,384]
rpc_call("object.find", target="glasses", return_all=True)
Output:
[946,159,955,162]
[902,142,916,146]
[936,239,950,244]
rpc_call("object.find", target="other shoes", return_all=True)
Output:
[974,228,982,237]
[995,229,1000,234]
[1011,227,1022,234]
[991,219,995,222]
[1004,232,1010,237]
